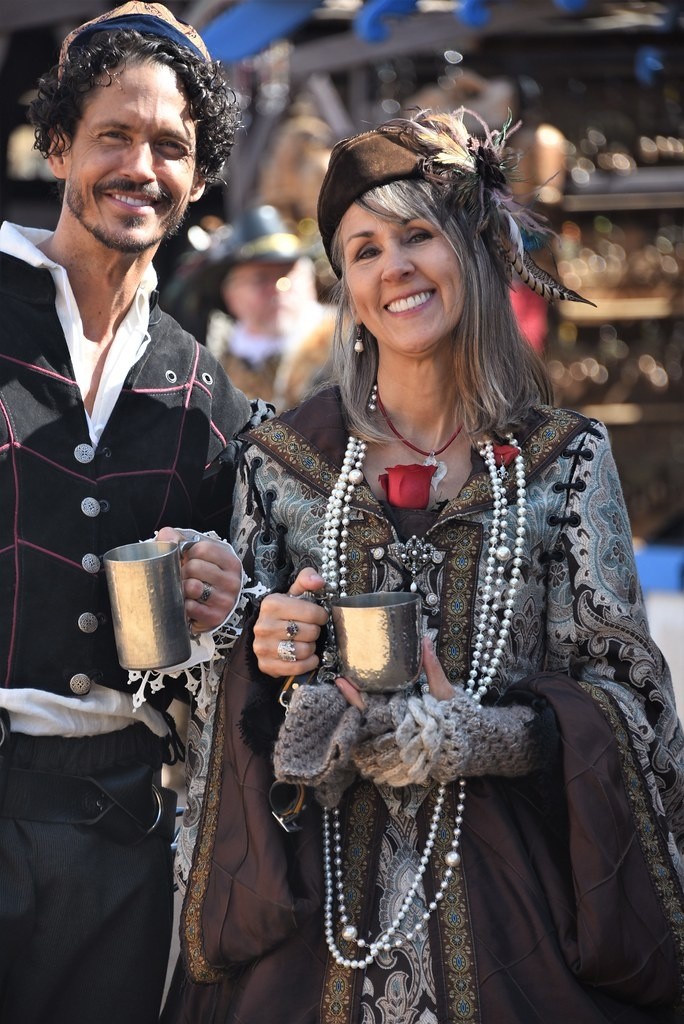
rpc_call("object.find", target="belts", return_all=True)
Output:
[0,761,177,848]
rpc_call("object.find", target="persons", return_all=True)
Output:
[185,111,684,1024]
[207,205,346,412]
[0,0,281,1024]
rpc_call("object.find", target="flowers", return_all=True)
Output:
[375,465,439,510]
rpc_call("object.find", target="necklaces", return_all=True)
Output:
[324,384,526,968]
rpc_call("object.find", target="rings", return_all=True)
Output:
[278,640,296,663]
[286,619,300,640]
[197,581,212,603]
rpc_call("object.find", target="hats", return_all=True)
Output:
[206,203,307,269]
[57,2,227,91]
[317,104,599,308]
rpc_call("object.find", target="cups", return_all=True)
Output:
[103,540,195,670]
[289,590,424,693]
